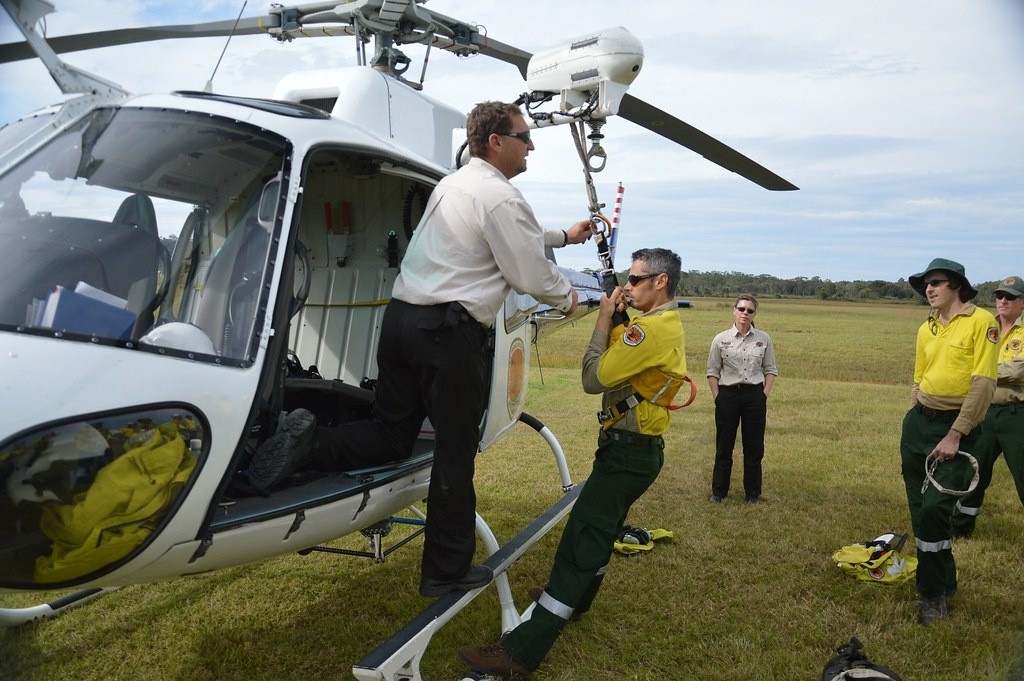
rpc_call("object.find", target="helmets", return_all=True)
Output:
[3,421,111,508]
[140,321,217,355]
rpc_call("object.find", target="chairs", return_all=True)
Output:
[194,175,311,362]
[111,194,171,342]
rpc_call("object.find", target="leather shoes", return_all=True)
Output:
[247,408,318,490]
[420,565,493,597]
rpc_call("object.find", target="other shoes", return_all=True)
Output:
[749,495,760,504]
[709,495,724,504]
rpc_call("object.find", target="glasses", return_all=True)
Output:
[627,273,668,286]
[736,307,756,314]
[923,279,951,288]
[487,131,530,144]
[995,292,1020,301]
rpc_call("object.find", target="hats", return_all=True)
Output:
[991,276,1024,296]
[909,258,978,304]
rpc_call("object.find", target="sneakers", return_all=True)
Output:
[459,631,531,680]
[530,585,581,620]
[922,593,947,627]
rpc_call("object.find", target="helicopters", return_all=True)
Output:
[1,0,799,680]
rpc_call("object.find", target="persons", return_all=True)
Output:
[899,258,1000,628]
[458,248,689,676]
[250,101,594,605]
[705,294,779,506]
[949,276,1024,541]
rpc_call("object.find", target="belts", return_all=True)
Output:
[921,406,953,417]
[598,427,661,446]
